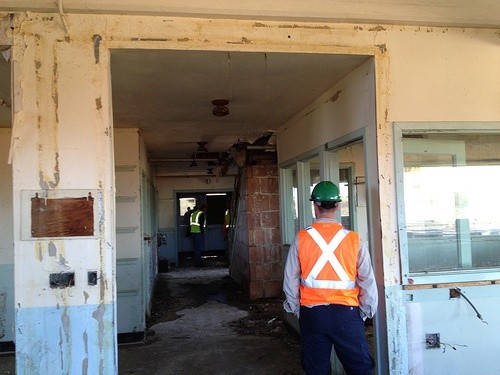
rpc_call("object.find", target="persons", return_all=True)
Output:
[284,181,379,375]
[185,205,231,269]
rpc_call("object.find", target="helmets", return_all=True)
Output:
[310,180,342,201]
[202,204,208,211]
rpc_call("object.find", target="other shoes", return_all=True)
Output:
[195,264,204,267]
